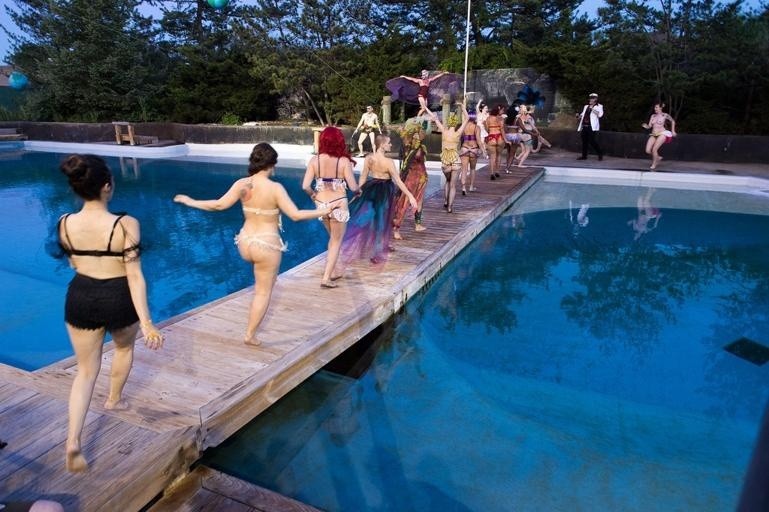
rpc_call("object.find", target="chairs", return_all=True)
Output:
[112,122,158,146]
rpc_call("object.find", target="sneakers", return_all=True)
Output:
[575,152,604,162]
[368,243,398,267]
[442,171,502,215]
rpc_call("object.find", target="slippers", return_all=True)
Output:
[330,275,344,281]
[320,282,340,289]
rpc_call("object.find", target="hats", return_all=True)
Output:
[588,92,600,100]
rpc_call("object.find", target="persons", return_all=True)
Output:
[351,105,383,158]
[641,100,678,173]
[48,153,166,468]
[400,69,450,116]
[357,135,418,263]
[300,126,360,288]
[576,93,605,163]
[392,117,429,240]
[423,102,470,212]
[565,200,591,240]
[175,142,340,344]
[625,186,663,241]
[461,101,542,194]
